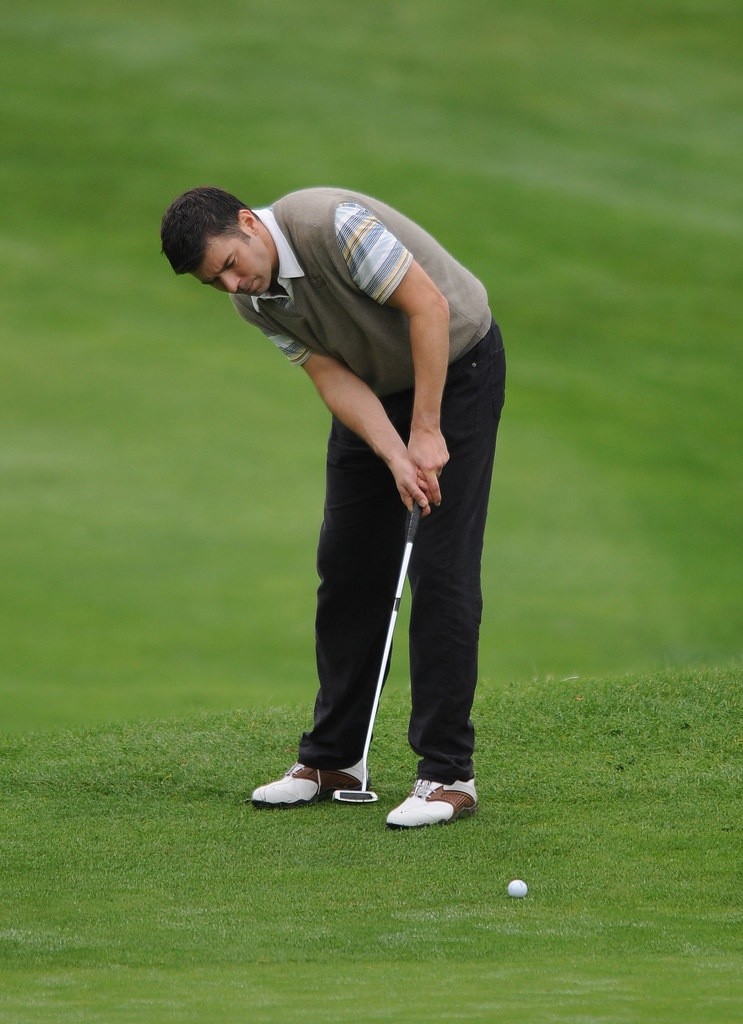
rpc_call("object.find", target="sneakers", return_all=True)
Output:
[387,777,478,827]
[251,759,369,808]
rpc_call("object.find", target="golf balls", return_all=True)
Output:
[507,879,528,899]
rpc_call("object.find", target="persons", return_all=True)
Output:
[159,186,506,830]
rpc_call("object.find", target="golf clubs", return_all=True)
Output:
[331,499,424,806]
